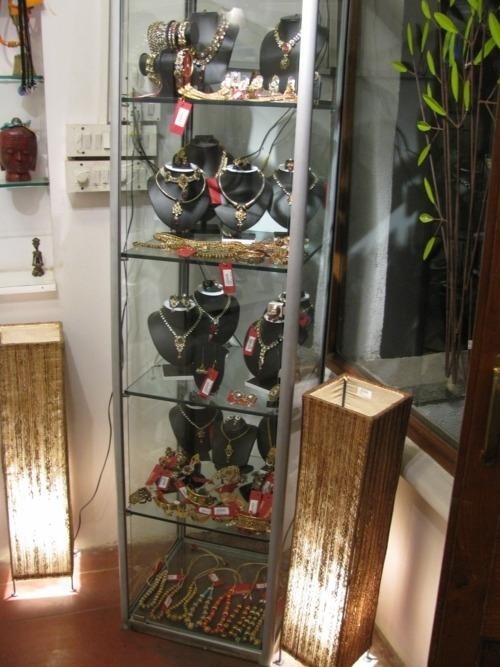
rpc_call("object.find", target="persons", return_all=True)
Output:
[0,116,39,183]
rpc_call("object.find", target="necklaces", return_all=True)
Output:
[219,422,250,465]
[159,308,203,359]
[273,25,300,72]
[278,293,315,316]
[138,540,268,649]
[272,170,320,208]
[174,146,229,179]
[218,171,265,228]
[264,418,276,469]
[176,405,219,445]
[143,10,232,100]
[152,168,207,222]
[132,232,308,269]
[194,294,230,341]
[255,316,284,372]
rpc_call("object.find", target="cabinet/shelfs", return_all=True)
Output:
[108,1,363,667]
[0,76,59,302]
